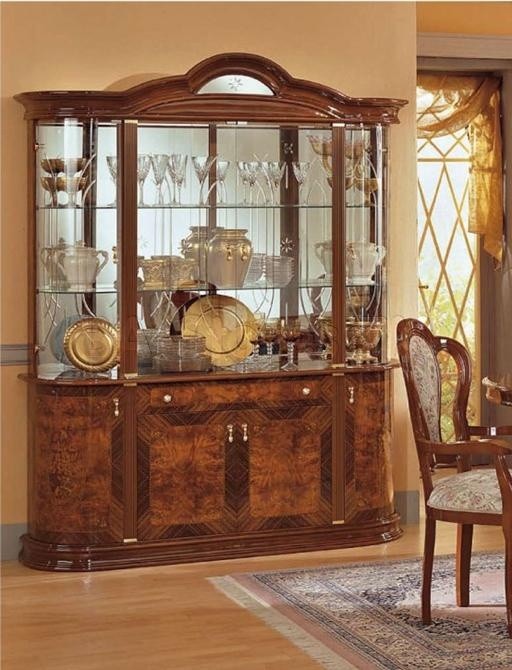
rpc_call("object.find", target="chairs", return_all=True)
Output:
[397,318,512,639]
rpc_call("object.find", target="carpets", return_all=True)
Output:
[207,548,512,670]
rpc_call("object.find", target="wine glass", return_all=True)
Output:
[251,286,382,369]
[41,136,380,207]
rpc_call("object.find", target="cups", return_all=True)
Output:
[142,255,200,290]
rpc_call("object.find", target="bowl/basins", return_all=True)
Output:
[146,333,211,372]
[244,253,265,285]
[264,255,295,286]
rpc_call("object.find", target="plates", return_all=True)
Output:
[50,318,83,367]
[181,295,259,369]
[136,330,170,364]
[64,315,121,375]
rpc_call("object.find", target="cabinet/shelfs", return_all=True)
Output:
[13,53,411,573]
